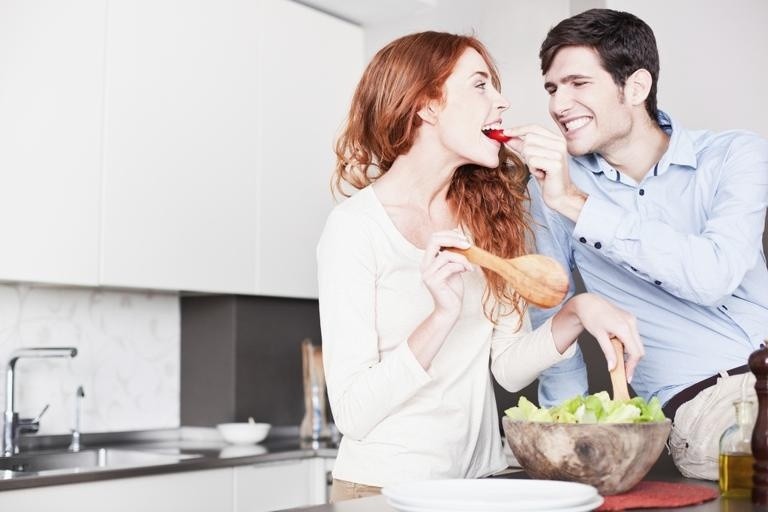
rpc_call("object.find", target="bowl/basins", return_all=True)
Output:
[502,415,672,493]
[218,444,268,458]
[218,422,272,444]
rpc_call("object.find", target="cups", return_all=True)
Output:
[297,338,331,439]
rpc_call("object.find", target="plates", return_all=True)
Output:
[383,476,605,512]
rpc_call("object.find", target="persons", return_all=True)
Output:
[505,9,767,481]
[316,31,645,504]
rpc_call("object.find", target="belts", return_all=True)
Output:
[662,366,750,419]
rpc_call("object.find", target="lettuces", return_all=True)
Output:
[504,391,667,422]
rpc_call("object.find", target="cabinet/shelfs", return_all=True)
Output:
[0,445,325,512]
[0,0,365,302]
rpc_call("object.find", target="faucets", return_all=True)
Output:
[3,348,78,458]
[69,387,85,453]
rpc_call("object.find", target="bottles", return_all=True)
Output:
[745,345,768,507]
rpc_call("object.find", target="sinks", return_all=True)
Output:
[0,444,181,482]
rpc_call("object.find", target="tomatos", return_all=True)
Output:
[485,130,511,142]
